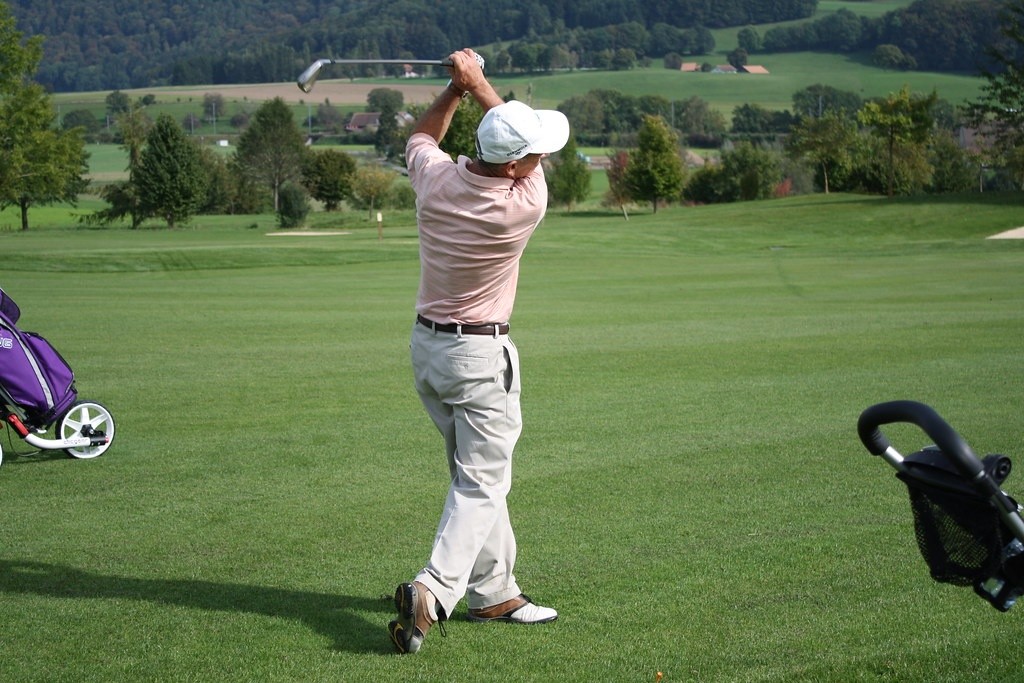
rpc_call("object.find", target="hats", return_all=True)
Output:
[473,100,570,165]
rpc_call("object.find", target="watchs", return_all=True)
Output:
[447,79,465,97]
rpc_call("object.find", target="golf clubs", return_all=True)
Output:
[297,58,455,94]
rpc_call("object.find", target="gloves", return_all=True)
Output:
[473,51,484,69]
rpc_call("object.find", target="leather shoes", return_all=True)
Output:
[388,582,439,655]
[467,594,558,623]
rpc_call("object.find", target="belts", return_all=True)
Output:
[416,313,510,336]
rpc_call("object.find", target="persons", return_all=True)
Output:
[389,49,570,653]
